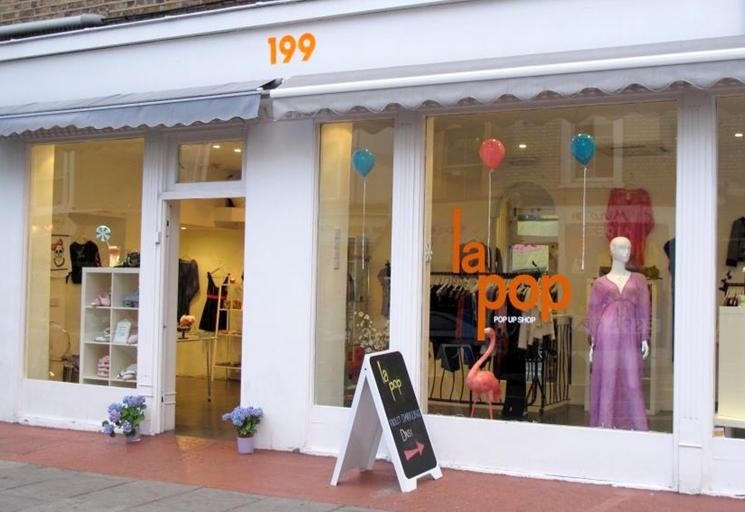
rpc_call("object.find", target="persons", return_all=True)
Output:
[583,236,651,431]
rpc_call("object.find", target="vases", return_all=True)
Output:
[124,424,141,442]
[236,436,255,454]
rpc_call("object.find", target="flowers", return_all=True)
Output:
[354,309,390,352]
[223,404,263,437]
[102,392,147,435]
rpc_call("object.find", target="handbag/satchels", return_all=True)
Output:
[124,252,140,266]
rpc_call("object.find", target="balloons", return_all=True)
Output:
[479,138,506,171]
[353,147,375,178]
[571,133,596,167]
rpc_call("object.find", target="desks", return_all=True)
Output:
[177,334,215,402]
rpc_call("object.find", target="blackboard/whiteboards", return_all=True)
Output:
[365,348,438,479]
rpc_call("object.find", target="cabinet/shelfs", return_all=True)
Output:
[212,280,242,386]
[79,265,143,387]
[346,256,370,380]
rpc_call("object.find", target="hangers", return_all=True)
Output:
[180,248,194,261]
[70,231,95,247]
[209,261,231,275]
[432,273,479,289]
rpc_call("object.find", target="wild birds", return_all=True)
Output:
[467,327,502,419]
